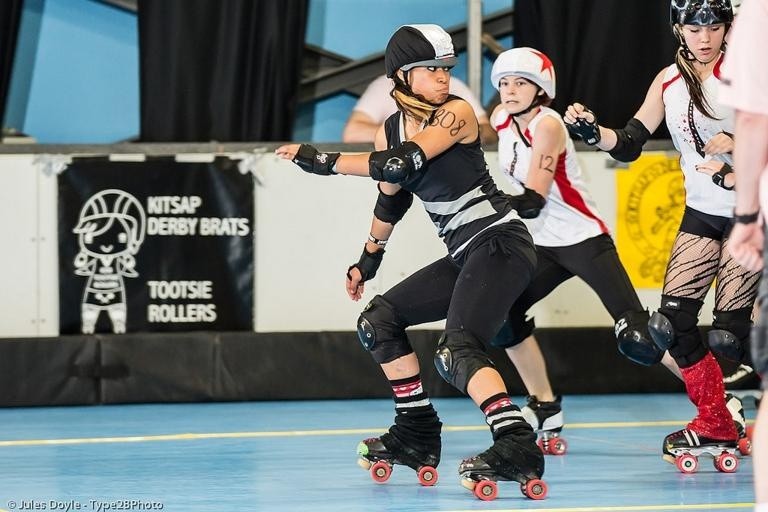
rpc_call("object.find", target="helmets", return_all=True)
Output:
[385,24,457,78]
[491,47,556,99]
[670,0,733,26]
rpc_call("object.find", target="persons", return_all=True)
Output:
[563,0,765,447]
[275,24,543,472]
[343,65,498,145]
[489,47,748,432]
[727,0,767,512]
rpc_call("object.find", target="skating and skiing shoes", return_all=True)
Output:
[663,393,752,473]
[357,393,441,486]
[459,395,566,500]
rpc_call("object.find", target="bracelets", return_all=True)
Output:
[367,232,388,246]
